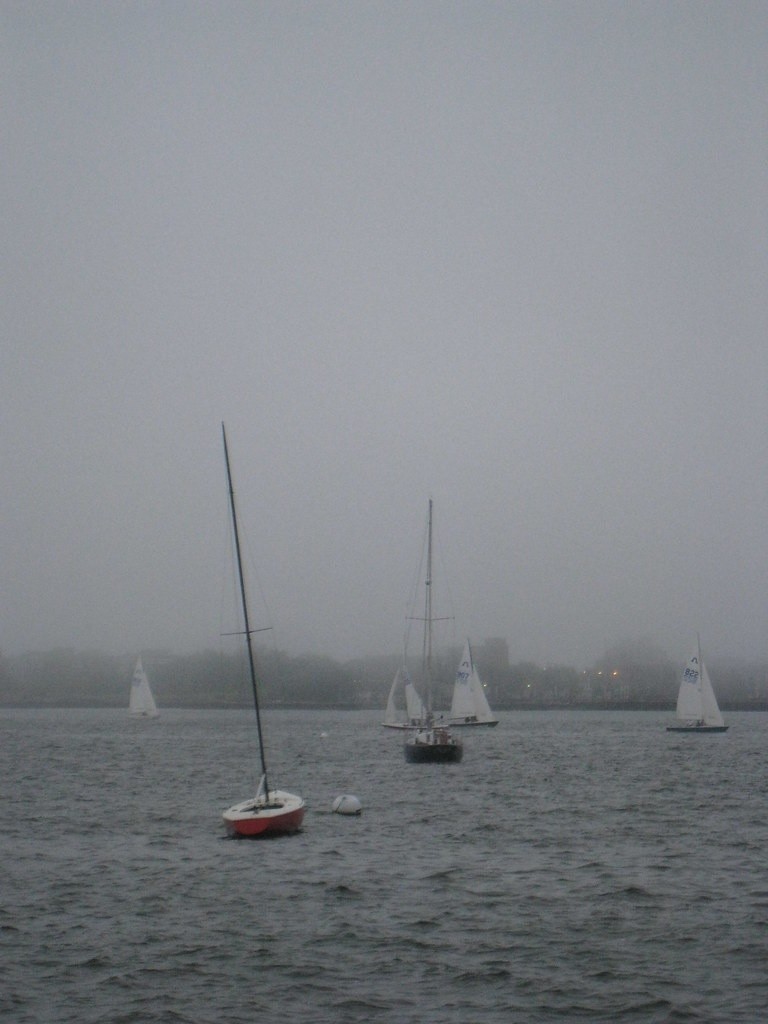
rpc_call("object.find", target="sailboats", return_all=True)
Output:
[125,655,161,720]
[665,632,730,732]
[220,419,308,837]
[380,634,450,731]
[401,499,464,766]
[445,628,502,728]
